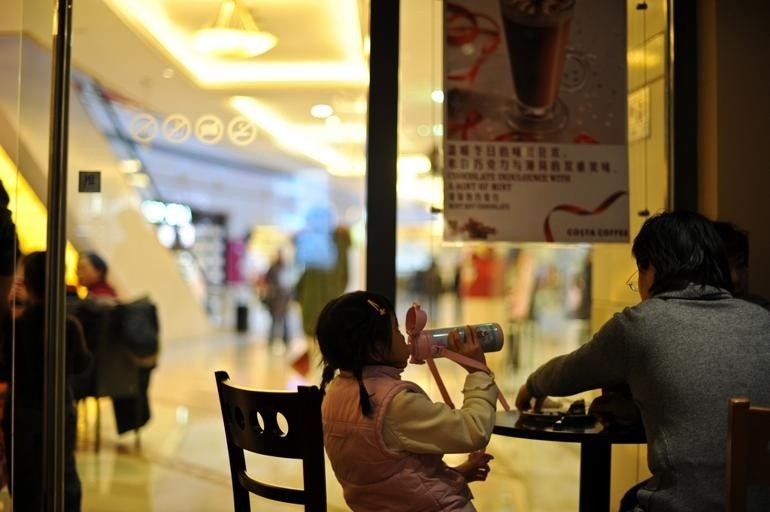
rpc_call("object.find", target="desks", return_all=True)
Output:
[487,403,654,512]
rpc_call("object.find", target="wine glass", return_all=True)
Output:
[499,0,577,137]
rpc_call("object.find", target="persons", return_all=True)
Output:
[0,251,96,510]
[514,207,768,512]
[260,245,292,348]
[75,252,120,302]
[315,291,499,512]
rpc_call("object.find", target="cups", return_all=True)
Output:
[405,302,504,365]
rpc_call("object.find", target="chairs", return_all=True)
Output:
[724,395,770,512]
[214,369,324,512]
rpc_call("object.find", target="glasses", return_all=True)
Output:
[625,266,641,293]
